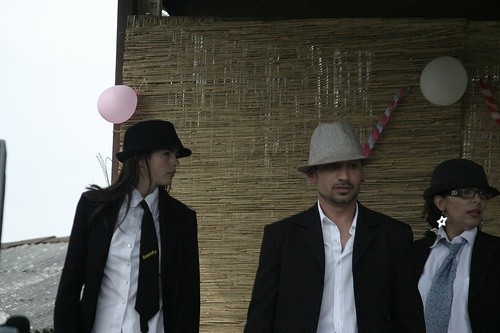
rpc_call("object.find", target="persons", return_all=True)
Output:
[415,158,500,333]
[52,118,201,332]
[5,314,30,332]
[243,120,426,333]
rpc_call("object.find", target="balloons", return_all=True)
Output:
[421,55,468,106]
[97,85,139,123]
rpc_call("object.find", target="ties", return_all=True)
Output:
[134,199,160,333]
[424,237,467,333]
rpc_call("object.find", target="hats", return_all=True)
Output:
[423,159,500,201]
[297,120,369,174]
[115,120,192,163]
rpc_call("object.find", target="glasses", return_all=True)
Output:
[438,187,494,199]
